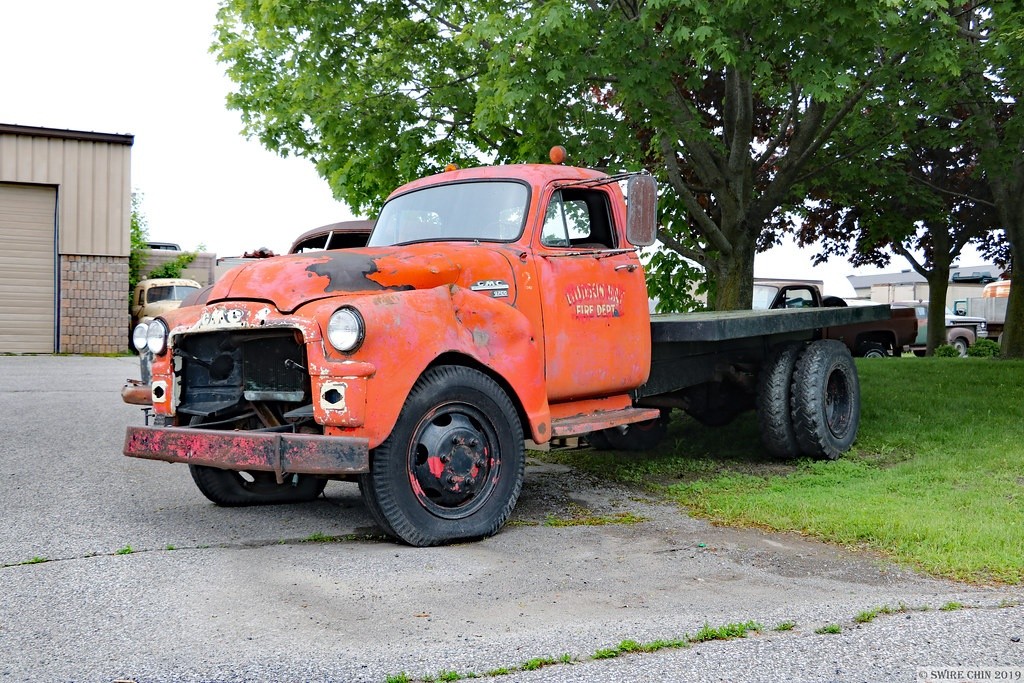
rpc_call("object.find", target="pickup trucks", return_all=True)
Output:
[892,302,977,360]
[752,281,918,359]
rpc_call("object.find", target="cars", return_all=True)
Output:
[919,302,989,337]
[132,280,201,337]
[139,243,180,251]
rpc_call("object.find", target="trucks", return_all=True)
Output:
[122,145,890,545]
[954,299,1009,341]
